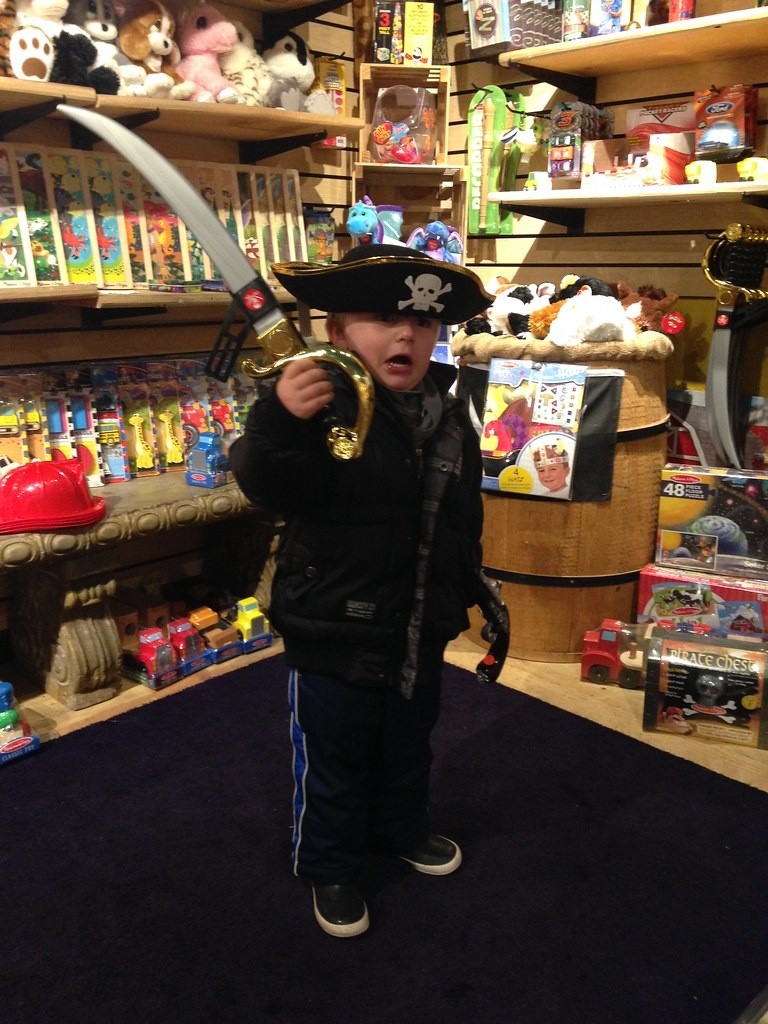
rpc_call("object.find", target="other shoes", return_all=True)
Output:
[311,882,370,938]
[392,832,462,876]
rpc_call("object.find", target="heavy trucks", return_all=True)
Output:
[579,617,649,688]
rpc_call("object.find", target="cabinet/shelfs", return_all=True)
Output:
[1,0,768,734]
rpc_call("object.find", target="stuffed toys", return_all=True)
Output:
[113,0,197,100]
[455,273,681,347]
[62,0,148,97]
[255,28,337,118]
[218,19,287,112]
[14,0,121,95]
[175,0,241,103]
[0,0,55,83]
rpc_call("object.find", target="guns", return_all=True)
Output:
[474,571,512,683]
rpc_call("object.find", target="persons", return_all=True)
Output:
[228,310,485,940]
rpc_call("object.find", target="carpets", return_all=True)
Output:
[1,627,767,1024]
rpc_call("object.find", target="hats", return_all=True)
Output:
[269,243,497,326]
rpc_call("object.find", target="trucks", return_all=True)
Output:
[123,593,272,675]
